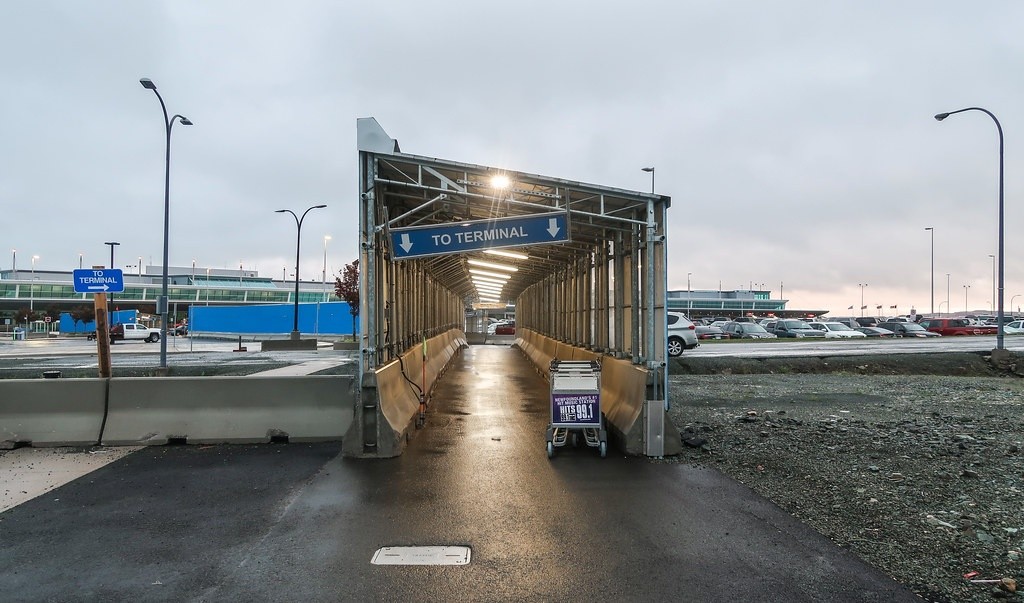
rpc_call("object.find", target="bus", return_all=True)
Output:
[504,305,515,318]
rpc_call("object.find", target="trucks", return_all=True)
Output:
[919,318,998,336]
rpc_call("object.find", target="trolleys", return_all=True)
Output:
[543,357,608,458]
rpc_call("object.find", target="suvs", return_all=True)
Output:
[169,324,189,336]
[668,312,700,358]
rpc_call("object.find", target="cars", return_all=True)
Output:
[960,314,1024,337]
[478,318,516,336]
[692,316,942,338]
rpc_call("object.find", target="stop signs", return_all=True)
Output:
[45,317,51,322]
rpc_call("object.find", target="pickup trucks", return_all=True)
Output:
[87,323,162,345]
[496,321,515,335]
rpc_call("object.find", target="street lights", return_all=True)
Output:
[987,254,996,314]
[1010,295,1022,316]
[30,256,40,312]
[963,285,970,315]
[925,226,935,317]
[322,236,332,302]
[858,282,868,317]
[78,253,287,283]
[275,204,328,331]
[136,74,194,365]
[641,167,655,194]
[945,273,950,313]
[688,273,693,319]
